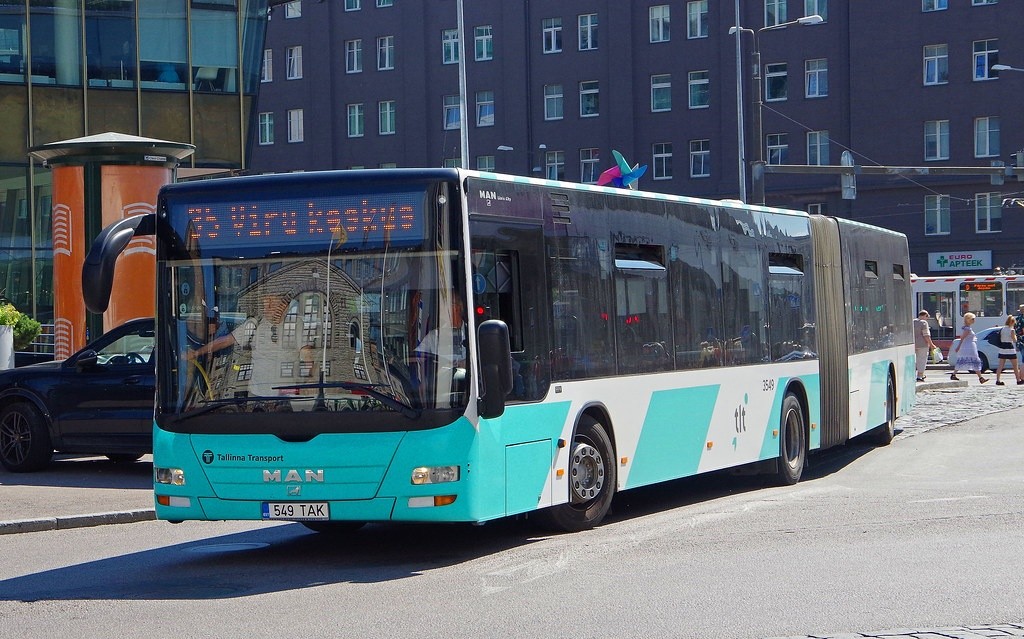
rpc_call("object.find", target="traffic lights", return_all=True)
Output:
[1010,151,1024,182]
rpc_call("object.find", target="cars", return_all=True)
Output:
[759,344,817,362]
[0,310,249,474]
[969,326,1024,374]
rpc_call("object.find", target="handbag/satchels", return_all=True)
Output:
[988,328,1001,348]
[930,347,943,364]
[238,319,260,365]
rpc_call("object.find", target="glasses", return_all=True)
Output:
[972,319,974,320]
[271,325,278,342]
[926,315,928,317]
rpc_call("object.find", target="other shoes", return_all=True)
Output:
[996,381,1004,385]
[979,377,988,384]
[951,374,959,380]
[917,375,926,381]
[1017,380,1024,384]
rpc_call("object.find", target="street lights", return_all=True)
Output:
[728,15,828,207]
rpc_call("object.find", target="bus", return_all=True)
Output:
[909,274,1023,360]
[0,236,54,319]
[82,168,916,537]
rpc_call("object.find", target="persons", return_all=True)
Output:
[951,312,989,384]
[184,284,315,412]
[413,297,467,381]
[914,310,936,382]
[996,304,1024,385]
